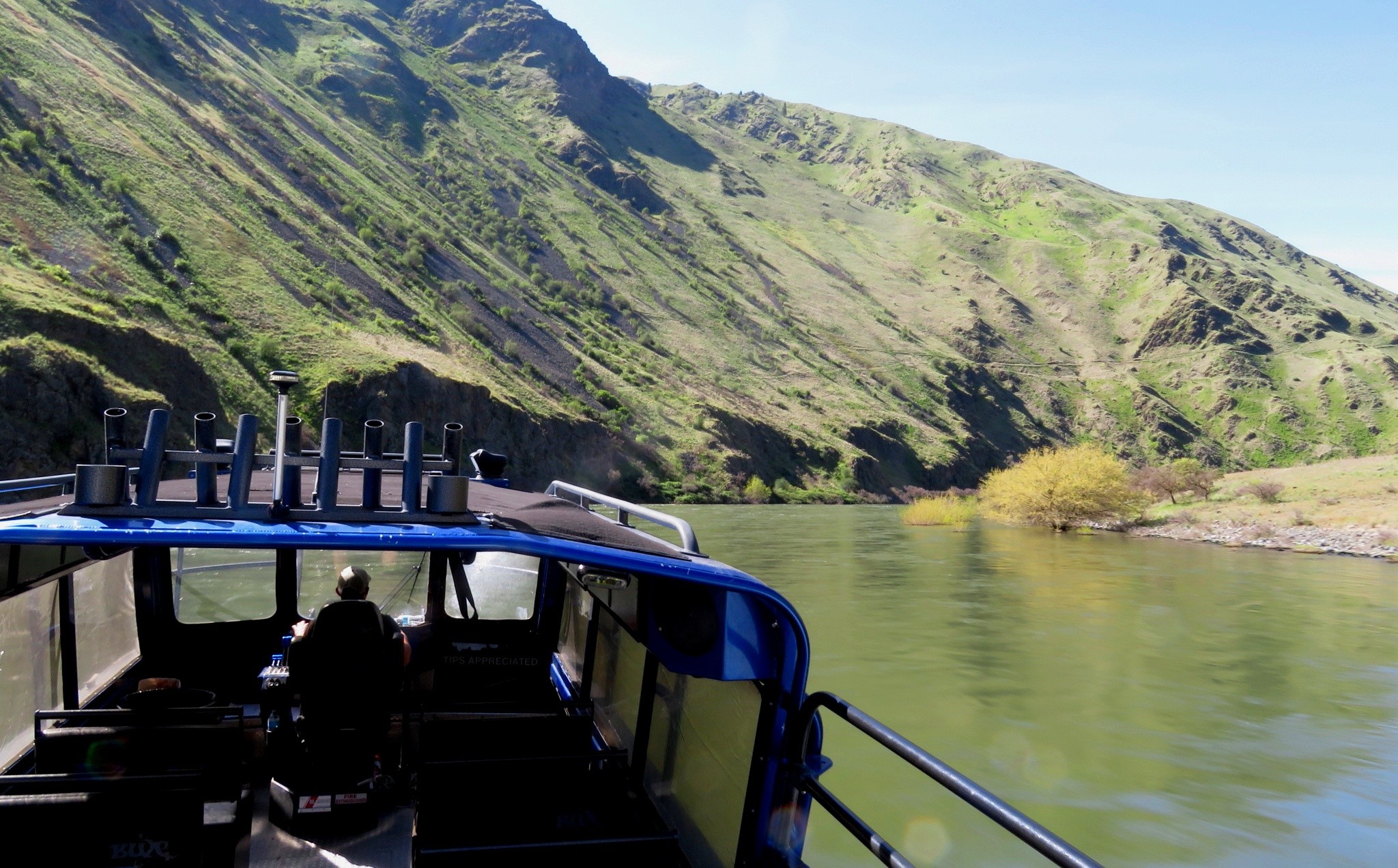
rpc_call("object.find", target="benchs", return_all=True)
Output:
[414,704,682,868]
[0,704,255,868]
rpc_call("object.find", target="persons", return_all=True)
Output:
[290,569,411,667]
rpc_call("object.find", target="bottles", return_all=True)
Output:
[374,753,381,776]
[267,710,279,731]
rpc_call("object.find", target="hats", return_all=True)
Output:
[337,566,373,595]
